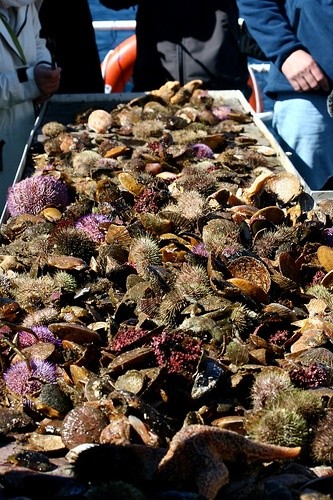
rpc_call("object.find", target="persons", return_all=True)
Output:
[98,0,254,103]
[0,0,61,221]
[237,0,333,191]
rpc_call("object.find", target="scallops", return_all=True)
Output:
[0,78,332,500]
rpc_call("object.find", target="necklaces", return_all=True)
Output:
[33,0,105,95]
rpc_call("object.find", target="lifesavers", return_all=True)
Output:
[99,33,263,115]
